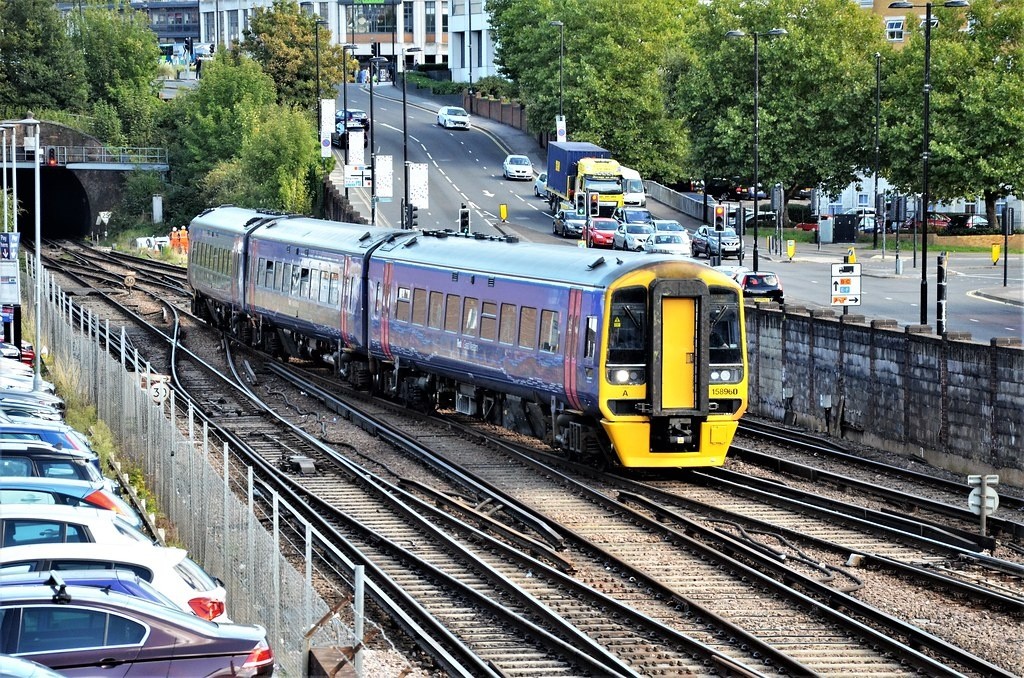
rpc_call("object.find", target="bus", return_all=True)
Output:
[159,43,197,71]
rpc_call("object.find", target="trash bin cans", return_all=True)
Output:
[819,214,834,244]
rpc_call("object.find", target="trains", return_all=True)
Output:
[187,203,749,473]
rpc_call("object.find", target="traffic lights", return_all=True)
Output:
[49,148,56,165]
[589,192,599,216]
[459,209,470,233]
[575,193,586,216]
[714,204,726,232]
[410,204,418,227]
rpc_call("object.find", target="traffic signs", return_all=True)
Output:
[831,263,862,306]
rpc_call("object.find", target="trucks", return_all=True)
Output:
[544,141,630,213]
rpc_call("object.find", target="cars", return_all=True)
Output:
[943,215,990,231]
[744,208,792,228]
[664,173,811,203]
[902,211,951,229]
[503,155,535,182]
[534,172,548,198]
[793,207,906,234]
[437,106,471,131]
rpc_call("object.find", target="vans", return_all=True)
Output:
[620,165,648,208]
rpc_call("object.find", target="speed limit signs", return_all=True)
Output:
[124,276,135,287]
[99,211,112,224]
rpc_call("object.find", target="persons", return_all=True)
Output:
[192,55,201,80]
[179,225,189,254]
[366,67,370,83]
[168,227,178,253]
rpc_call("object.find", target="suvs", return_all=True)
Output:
[552,206,690,256]
[690,225,745,260]
[0,304,274,678]
[712,266,784,304]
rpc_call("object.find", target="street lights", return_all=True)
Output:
[0,124,19,305]
[369,56,388,225]
[872,53,887,251]
[1,128,8,233]
[17,118,43,390]
[342,44,359,200]
[315,21,329,142]
[549,20,565,143]
[402,47,424,230]
[889,0,969,325]
[724,27,788,271]
